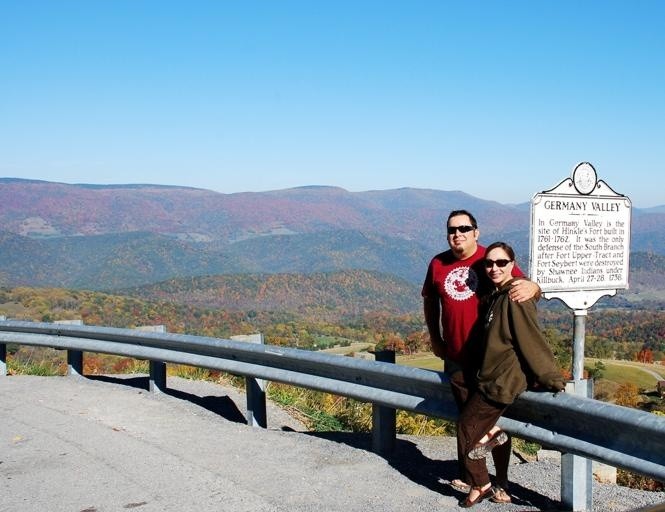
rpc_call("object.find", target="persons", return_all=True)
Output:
[421,210,542,504]
[443,242,566,507]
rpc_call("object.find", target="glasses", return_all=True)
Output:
[447,226,474,234]
[483,259,512,267]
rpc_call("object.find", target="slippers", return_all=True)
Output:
[467,430,509,460]
[451,470,512,507]
